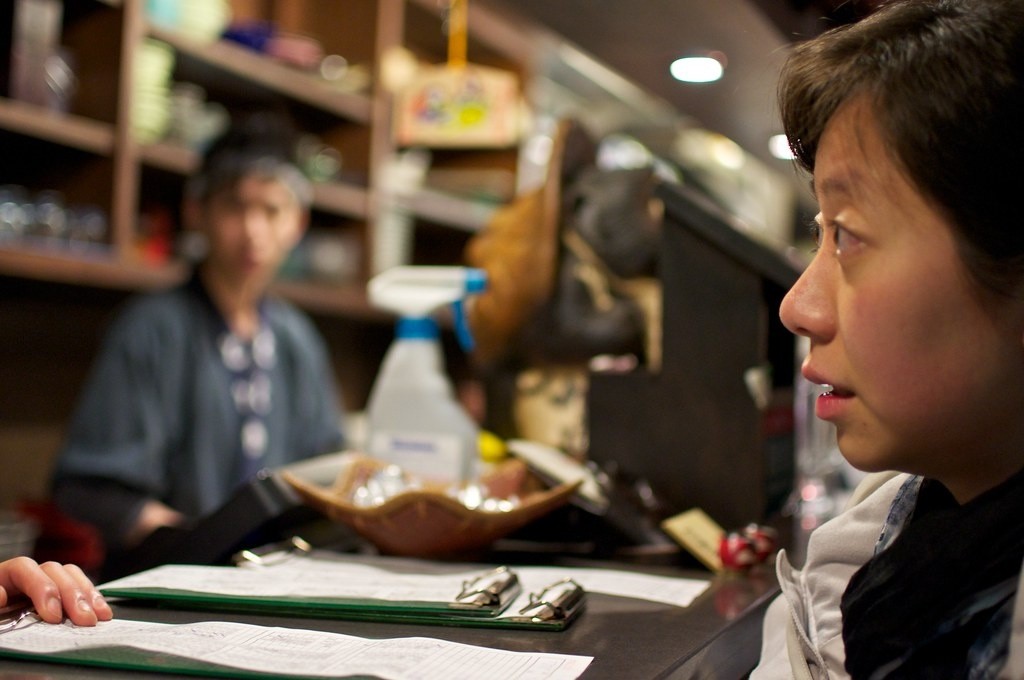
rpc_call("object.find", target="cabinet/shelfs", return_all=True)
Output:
[0,1,544,327]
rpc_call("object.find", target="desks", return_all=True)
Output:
[1,528,792,680]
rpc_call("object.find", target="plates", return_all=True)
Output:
[257,462,583,555]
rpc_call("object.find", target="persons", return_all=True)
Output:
[57,131,346,547]
[0,556,114,627]
[748,0,1024,680]
